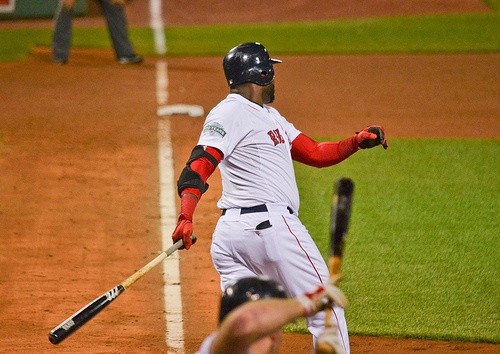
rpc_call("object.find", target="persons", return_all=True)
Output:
[171,40,388,353]
[195,276,348,354]
[49,0,144,66]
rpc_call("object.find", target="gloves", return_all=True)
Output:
[172,213,197,250]
[314,325,344,354]
[295,274,350,317]
[355,125,388,158]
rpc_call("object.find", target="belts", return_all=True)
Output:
[222,204,293,229]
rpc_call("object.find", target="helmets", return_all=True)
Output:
[223,42,282,89]
[217,276,287,327]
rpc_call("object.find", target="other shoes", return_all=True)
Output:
[51,51,66,65]
[118,55,144,65]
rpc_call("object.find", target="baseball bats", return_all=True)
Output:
[319,178,356,354]
[46,236,198,344]
